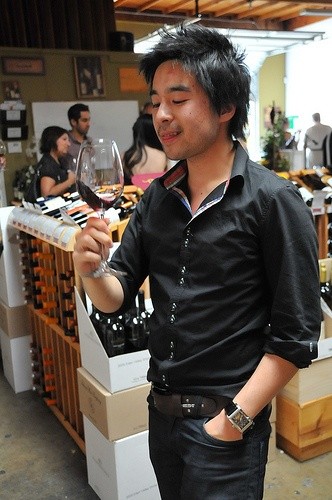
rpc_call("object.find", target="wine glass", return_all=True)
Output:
[75,138,128,279]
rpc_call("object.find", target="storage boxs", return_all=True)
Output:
[0,206,34,394]
[76,283,332,499]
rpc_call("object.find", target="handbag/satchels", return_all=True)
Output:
[23,157,61,204]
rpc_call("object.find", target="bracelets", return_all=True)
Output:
[74,260,106,279]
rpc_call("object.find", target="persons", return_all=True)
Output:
[58,104,96,194]
[34,126,77,204]
[71,23,321,500]
[302,113,331,169]
[122,102,170,194]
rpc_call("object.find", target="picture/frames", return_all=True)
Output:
[73,53,107,99]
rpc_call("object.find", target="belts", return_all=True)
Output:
[151,384,232,417]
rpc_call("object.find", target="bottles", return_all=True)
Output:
[288,178,314,206]
[16,232,77,407]
[20,186,140,231]
[105,316,126,358]
[315,168,332,188]
[125,301,144,352]
[138,291,151,350]
[305,174,332,204]
[320,264,332,321]
[100,313,117,346]
[89,304,104,338]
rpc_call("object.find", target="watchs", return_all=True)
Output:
[224,401,255,435]
